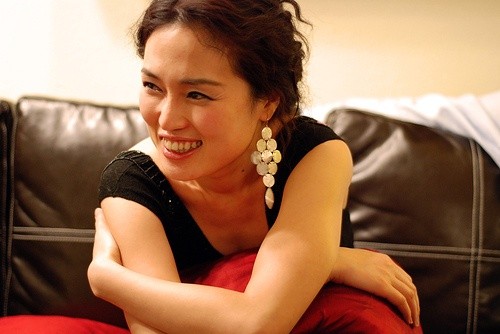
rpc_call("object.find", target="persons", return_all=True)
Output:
[87,1,419,334]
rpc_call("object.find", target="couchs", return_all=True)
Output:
[0,90,500,334]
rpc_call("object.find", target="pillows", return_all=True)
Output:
[2,97,153,330]
[327,108,499,334]
